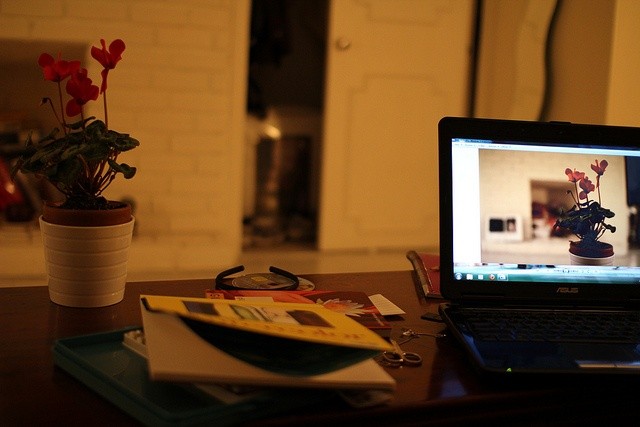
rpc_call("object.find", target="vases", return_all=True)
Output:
[568,256,615,266]
[40,202,133,225]
[568,241,614,256]
[39,218,133,308]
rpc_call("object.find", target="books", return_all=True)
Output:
[121,290,396,407]
[406,250,441,299]
[203,286,390,337]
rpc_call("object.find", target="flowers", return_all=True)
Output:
[14,38,140,209]
[552,161,617,245]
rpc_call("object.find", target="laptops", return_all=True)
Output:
[438,116,637,389]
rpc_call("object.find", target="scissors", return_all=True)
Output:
[383,335,421,364]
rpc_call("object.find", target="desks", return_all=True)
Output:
[3,271,489,426]
